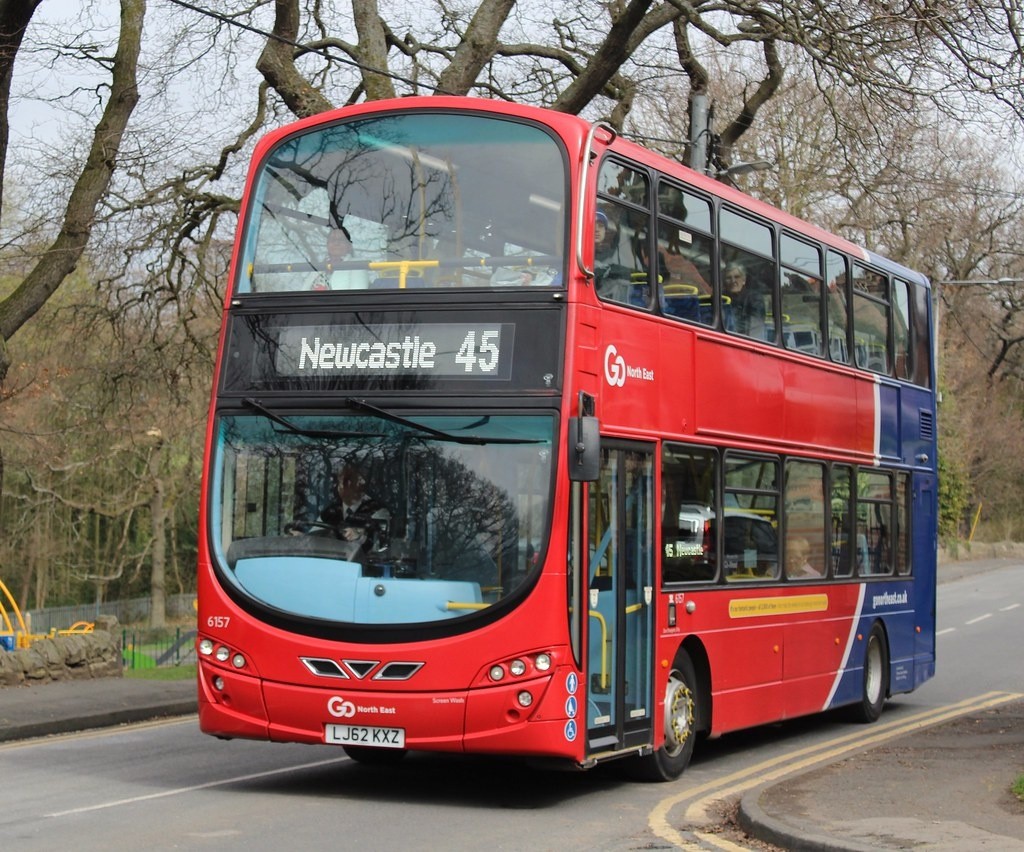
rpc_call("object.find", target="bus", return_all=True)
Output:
[194,94,938,787]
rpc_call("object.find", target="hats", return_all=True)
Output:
[595,206,608,229]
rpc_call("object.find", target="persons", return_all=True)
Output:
[280,457,390,554]
[549,207,632,305]
[301,226,369,290]
[721,262,768,342]
[765,536,821,579]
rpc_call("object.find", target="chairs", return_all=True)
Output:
[371,267,888,381]
